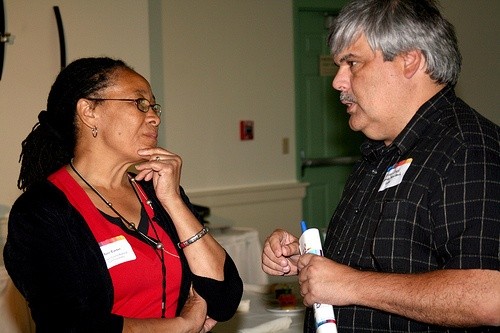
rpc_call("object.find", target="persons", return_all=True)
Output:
[3,57,244,333]
[262,0,500,333]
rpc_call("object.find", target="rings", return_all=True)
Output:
[156,156,160,161]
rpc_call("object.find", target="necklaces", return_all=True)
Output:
[69,157,183,318]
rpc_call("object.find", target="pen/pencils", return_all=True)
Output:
[300,218,306,234]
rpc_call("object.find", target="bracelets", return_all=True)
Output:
[177,227,209,249]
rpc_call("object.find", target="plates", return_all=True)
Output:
[268,305,306,312]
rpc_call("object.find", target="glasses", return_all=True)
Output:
[89,98,161,116]
